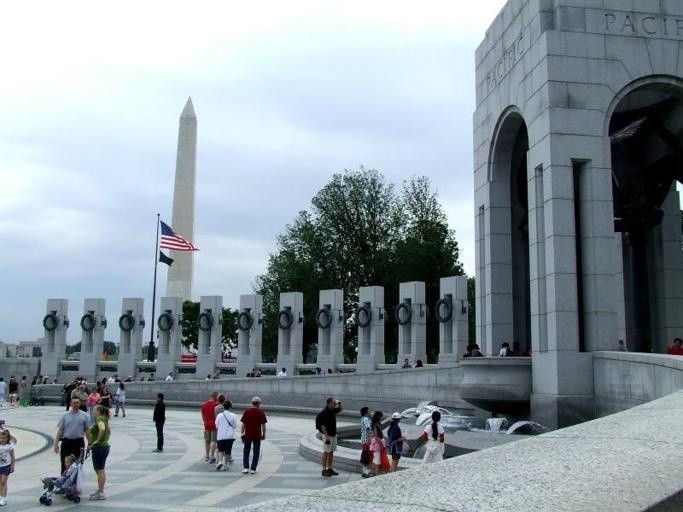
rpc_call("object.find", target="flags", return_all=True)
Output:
[159,220,199,251]
[157,252,175,266]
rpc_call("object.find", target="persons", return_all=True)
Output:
[420,411,445,466]
[213,372,220,379]
[462,341,521,357]
[385,412,407,473]
[214,393,234,462]
[318,397,343,477]
[152,393,166,453]
[164,372,176,382]
[401,359,412,368]
[0,372,133,507]
[245,368,262,377]
[205,374,212,380]
[239,396,267,474]
[666,337,683,355]
[370,411,390,476]
[277,368,286,377]
[215,400,237,472]
[146,373,155,380]
[359,407,375,478]
[202,392,219,463]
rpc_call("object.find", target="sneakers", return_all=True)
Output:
[321,468,338,476]
[362,472,376,478]
[241,468,257,474]
[152,448,162,453]
[88,488,106,501]
[204,455,233,470]
[0,497,7,505]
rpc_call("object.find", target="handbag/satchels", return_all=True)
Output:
[379,448,390,470]
[316,411,322,432]
[360,450,374,465]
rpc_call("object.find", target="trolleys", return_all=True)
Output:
[29,389,44,407]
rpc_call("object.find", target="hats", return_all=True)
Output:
[392,412,401,418]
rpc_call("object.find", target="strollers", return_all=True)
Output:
[40,448,91,507]
